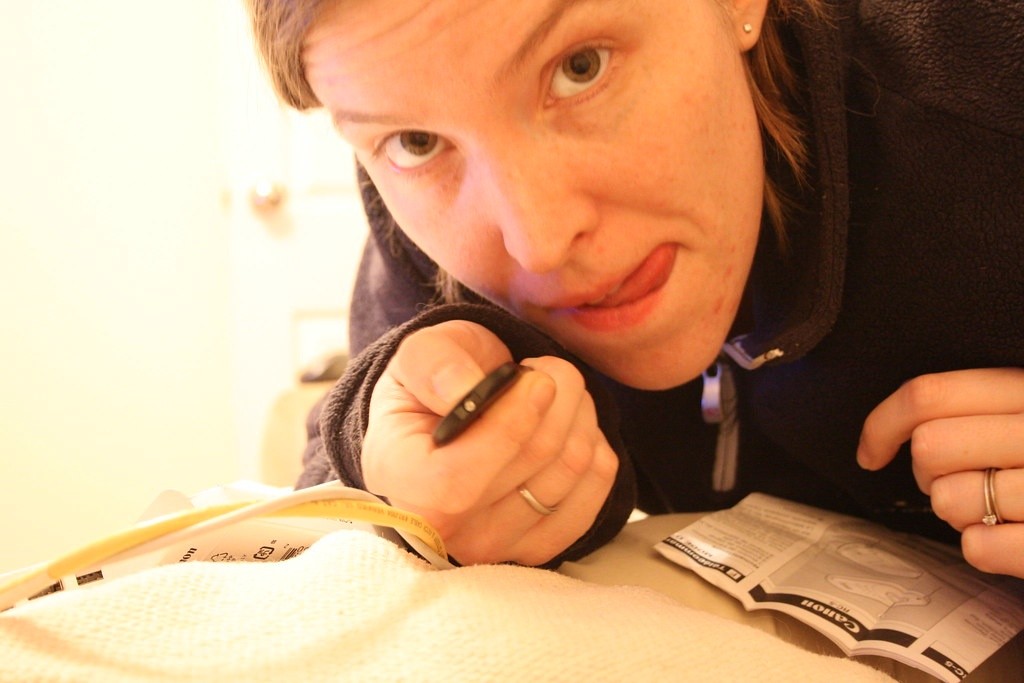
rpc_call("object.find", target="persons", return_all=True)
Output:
[241,0,1024,578]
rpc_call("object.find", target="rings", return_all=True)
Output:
[517,485,559,516]
[982,468,1004,526]
